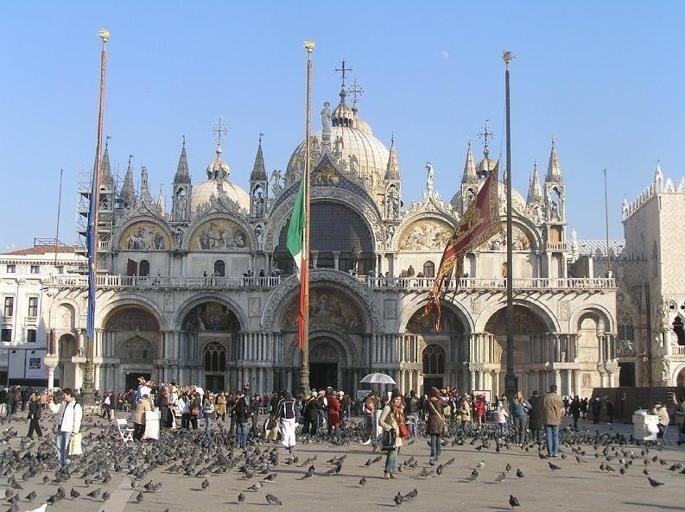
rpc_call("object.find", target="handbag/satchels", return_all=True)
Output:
[191,405,199,416]
[443,420,449,433]
[381,428,396,452]
[67,432,82,456]
[202,404,215,414]
[398,423,410,437]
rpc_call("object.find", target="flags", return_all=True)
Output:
[414,157,506,333]
[84,157,94,337]
[285,170,306,357]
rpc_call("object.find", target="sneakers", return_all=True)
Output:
[546,454,560,457]
[383,472,397,480]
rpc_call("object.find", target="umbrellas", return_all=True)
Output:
[359,372,398,386]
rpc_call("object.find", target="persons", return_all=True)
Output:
[672,392,685,446]
[361,383,615,480]
[156,273,161,284]
[651,399,670,438]
[204,271,208,286]
[367,264,472,288]
[93,372,355,449]
[241,268,280,287]
[0,383,83,470]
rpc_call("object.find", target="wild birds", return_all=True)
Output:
[0,411,685,512]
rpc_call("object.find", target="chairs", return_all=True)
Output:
[116,418,135,442]
